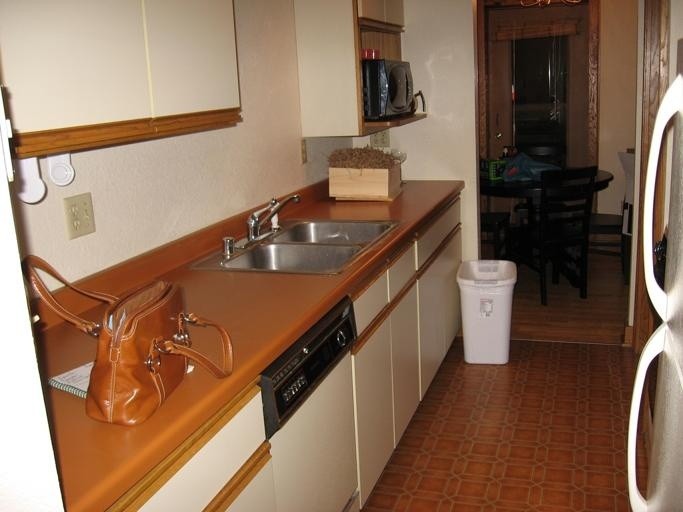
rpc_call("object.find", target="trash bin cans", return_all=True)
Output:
[453,259,520,367]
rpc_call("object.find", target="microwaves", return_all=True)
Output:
[362,61,412,122]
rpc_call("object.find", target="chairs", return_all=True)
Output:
[479,206,510,255]
[617,149,639,283]
[497,163,598,304]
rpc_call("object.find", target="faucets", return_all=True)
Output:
[246,192,301,241]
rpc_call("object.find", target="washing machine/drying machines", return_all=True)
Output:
[515,108,564,208]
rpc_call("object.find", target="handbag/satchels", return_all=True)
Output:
[19,249,236,427]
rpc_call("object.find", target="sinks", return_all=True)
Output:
[185,242,367,277]
[263,215,401,243]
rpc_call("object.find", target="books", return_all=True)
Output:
[46,355,195,399]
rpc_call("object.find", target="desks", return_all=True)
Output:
[480,169,615,264]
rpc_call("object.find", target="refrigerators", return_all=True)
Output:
[622,74,683,510]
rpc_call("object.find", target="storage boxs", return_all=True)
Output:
[326,158,403,199]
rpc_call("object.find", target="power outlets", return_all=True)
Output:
[61,192,97,242]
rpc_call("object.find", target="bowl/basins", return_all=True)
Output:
[360,48,380,60]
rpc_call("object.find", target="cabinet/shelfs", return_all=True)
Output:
[294,0,430,141]
[105,383,286,510]
[1,3,244,158]
[414,196,463,404]
[350,239,419,511]
